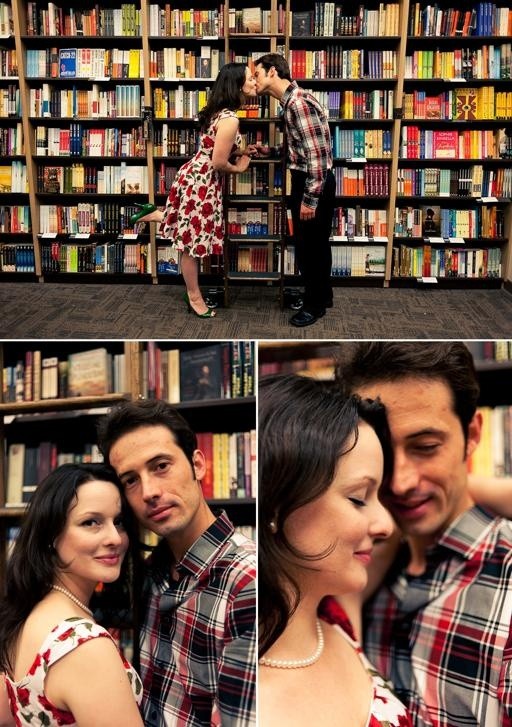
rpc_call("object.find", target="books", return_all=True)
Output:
[209,243,296,276]
[396,165,512,199]
[405,44,511,80]
[289,1,400,37]
[0,121,24,156]
[408,0,512,36]
[148,3,224,37]
[25,47,142,78]
[29,80,145,118]
[0,442,105,509]
[229,7,272,33]
[0,243,36,274]
[0,161,28,194]
[399,124,512,160]
[38,202,147,234]
[35,162,148,193]
[154,124,286,158]
[324,89,395,120]
[225,206,292,237]
[0,85,21,117]
[331,244,386,275]
[42,241,151,273]
[393,205,505,239]
[229,158,295,196]
[188,429,256,500]
[148,46,225,79]
[150,85,270,119]
[339,129,392,159]
[0,2,14,37]
[401,85,512,122]
[338,163,389,196]
[0,47,18,79]
[288,43,398,79]
[26,2,143,36]
[152,163,180,194]
[0,204,33,232]
[393,244,503,278]
[2,346,124,403]
[139,341,253,398]
[154,243,182,273]
[338,206,388,238]
[34,122,144,157]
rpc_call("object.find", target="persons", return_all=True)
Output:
[130,62,258,318]
[257,373,512,726]
[102,399,257,727]
[332,342,512,725]
[250,52,338,326]
[0,462,147,726]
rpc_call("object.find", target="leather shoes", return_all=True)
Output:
[289,305,326,327]
[325,298,333,308]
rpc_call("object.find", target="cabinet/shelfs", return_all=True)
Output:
[258,339,512,480]
[0,0,511,288]
[0,340,257,597]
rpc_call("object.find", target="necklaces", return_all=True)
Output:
[46,583,95,618]
[255,616,323,667]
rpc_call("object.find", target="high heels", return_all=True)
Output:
[181,290,216,318]
[129,203,157,228]
